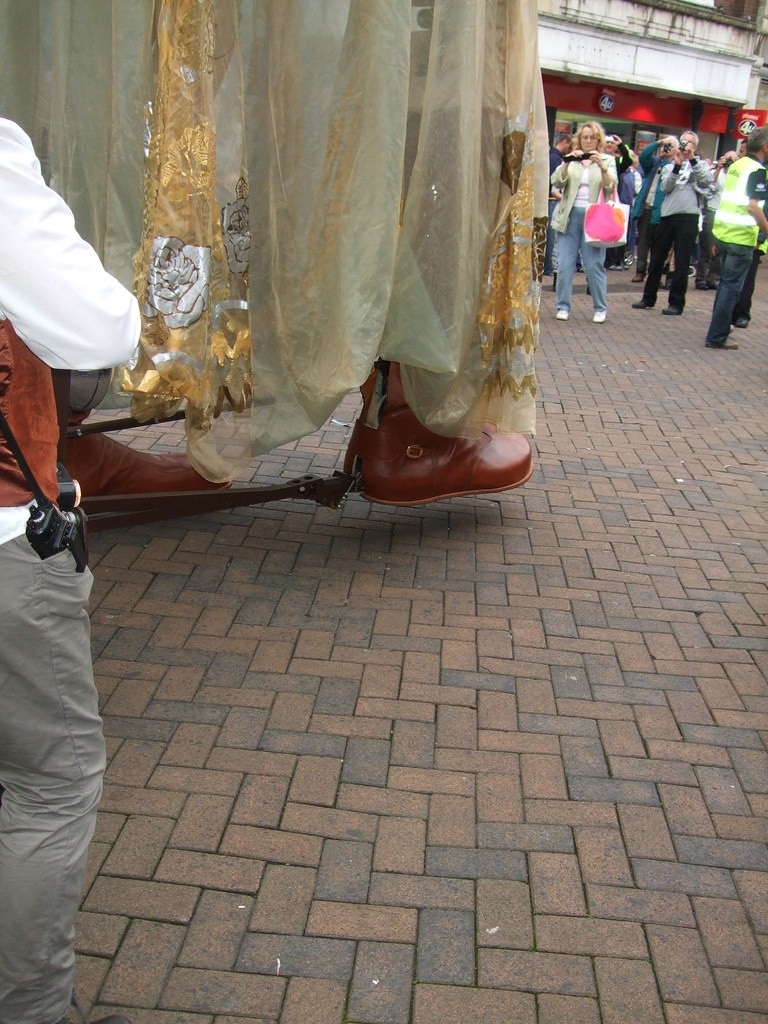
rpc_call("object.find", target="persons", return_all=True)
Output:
[632,130,713,315]
[1,1,550,504]
[705,126,768,349]
[0,117,143,1024]
[630,137,680,282]
[731,198,768,327]
[544,132,645,295]
[550,120,618,323]
[659,138,749,289]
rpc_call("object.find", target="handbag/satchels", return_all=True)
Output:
[584,180,630,248]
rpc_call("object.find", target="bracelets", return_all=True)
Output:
[602,169,608,173]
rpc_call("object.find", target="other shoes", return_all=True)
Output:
[556,310,568,320]
[87,1015,131,1024]
[632,273,644,282]
[708,279,719,289]
[606,258,634,270]
[705,339,738,349]
[662,305,682,315]
[696,283,709,289]
[633,300,654,308]
[665,278,672,289]
[732,315,748,328]
[728,325,734,337]
[593,310,606,322]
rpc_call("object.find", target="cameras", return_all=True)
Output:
[604,136,614,142]
[724,157,735,170]
[679,140,689,150]
[664,143,673,152]
